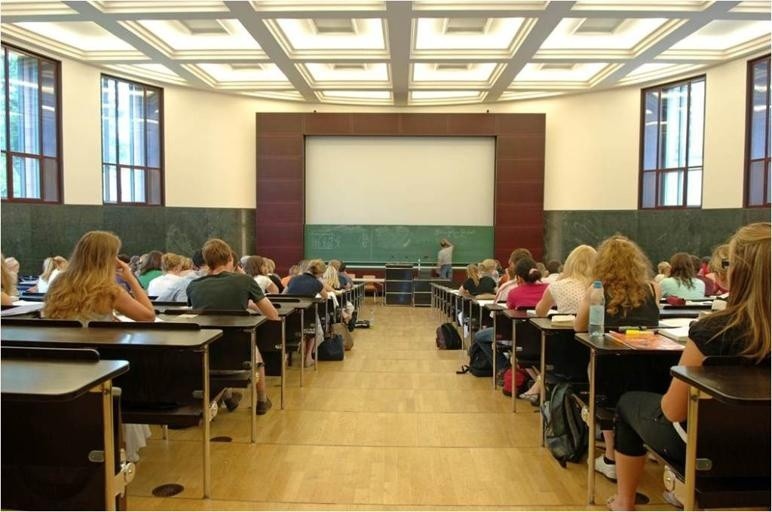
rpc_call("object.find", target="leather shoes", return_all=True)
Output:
[519,394,537,403]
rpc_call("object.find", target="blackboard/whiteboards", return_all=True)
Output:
[303,224,494,266]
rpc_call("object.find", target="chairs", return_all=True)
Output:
[348,274,384,306]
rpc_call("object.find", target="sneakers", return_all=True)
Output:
[225,392,242,411]
[257,399,271,415]
[663,491,682,507]
[595,454,616,481]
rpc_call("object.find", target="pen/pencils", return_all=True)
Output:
[617,325,656,337]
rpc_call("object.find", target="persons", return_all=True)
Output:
[1,231,352,463]
[457,222,771,511]
[437,239,454,280]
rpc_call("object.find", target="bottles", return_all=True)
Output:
[492,270,499,292]
[589,280,605,338]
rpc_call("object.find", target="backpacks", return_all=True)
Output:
[318,323,353,360]
[541,383,589,467]
[456,341,511,377]
[437,323,462,350]
[503,368,528,397]
